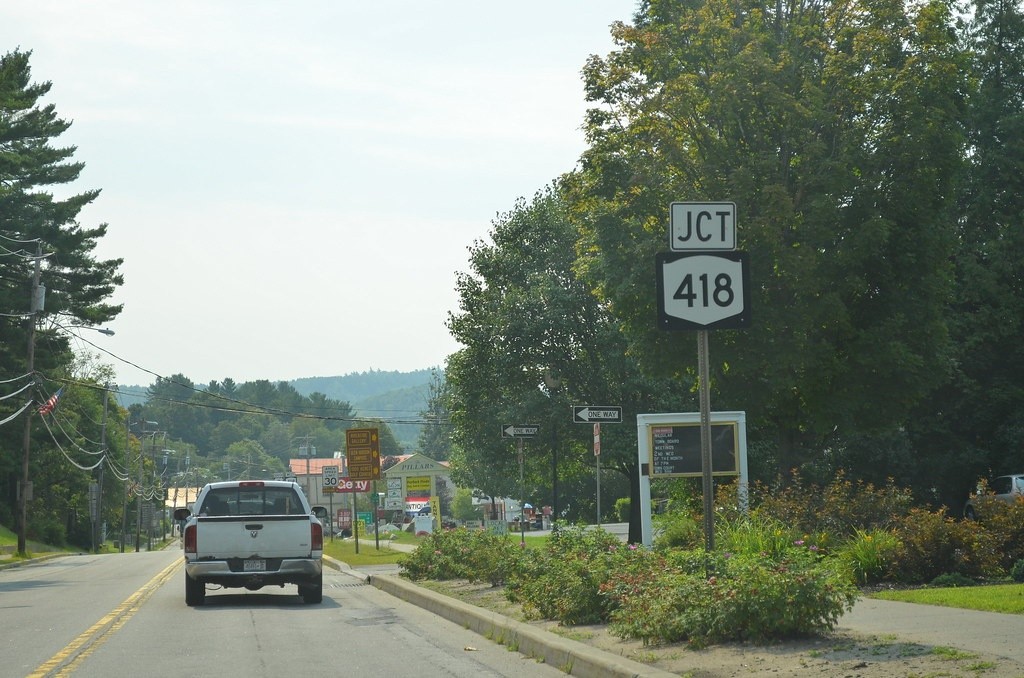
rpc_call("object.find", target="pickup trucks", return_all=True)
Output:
[174,480,328,605]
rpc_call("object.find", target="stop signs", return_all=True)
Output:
[593,423,601,455]
[517,440,523,464]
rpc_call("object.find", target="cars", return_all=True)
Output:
[965,474,1024,518]
[441,520,457,530]
[324,527,352,538]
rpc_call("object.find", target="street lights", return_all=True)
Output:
[19,326,117,556]
[120,422,158,550]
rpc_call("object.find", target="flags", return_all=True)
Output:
[40,388,62,414]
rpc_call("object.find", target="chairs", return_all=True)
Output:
[274,498,293,513]
[209,501,230,514]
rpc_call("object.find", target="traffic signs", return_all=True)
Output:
[572,405,622,424]
[500,424,540,438]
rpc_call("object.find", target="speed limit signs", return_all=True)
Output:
[322,466,338,492]
